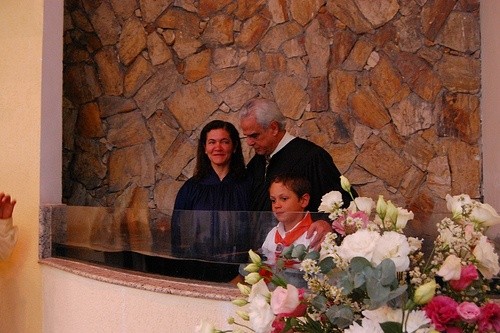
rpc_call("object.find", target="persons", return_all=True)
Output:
[239,97,359,253]
[171,119,278,287]
[259,172,338,277]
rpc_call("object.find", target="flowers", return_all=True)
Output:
[195,174,500,333]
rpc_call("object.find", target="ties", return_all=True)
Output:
[274,212,312,263]
[263,158,271,182]
